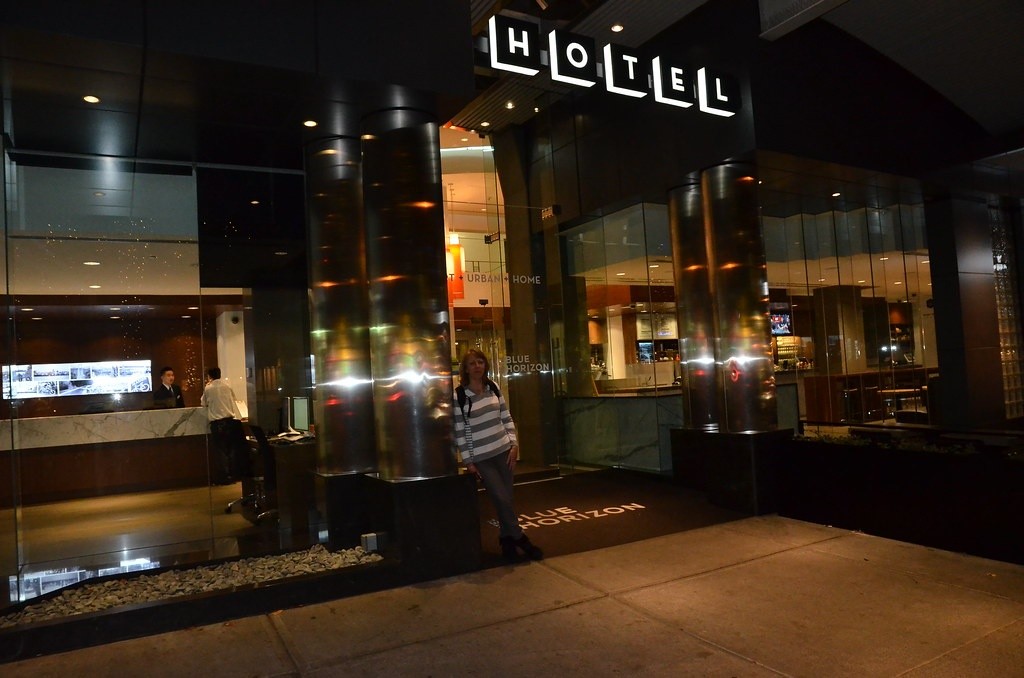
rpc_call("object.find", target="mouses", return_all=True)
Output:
[278,441,290,445]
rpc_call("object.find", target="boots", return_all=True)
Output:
[499,535,523,562]
[515,532,544,560]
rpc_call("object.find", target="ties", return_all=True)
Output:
[168,386,172,393]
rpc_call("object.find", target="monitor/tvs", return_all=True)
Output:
[290,397,310,441]
[276,397,300,437]
[771,313,792,336]
[770,314,794,336]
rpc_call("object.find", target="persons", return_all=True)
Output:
[153,367,185,409]
[200,367,237,485]
[452,349,543,561]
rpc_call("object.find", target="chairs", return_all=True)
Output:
[222,419,279,528]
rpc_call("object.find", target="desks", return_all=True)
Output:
[877,388,920,412]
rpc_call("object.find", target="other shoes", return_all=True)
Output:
[214,475,234,485]
